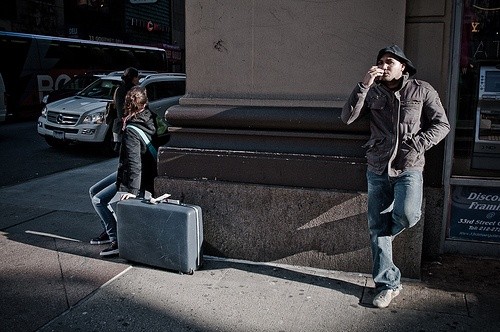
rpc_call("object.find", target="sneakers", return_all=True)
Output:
[99,241,119,255]
[373,287,400,308]
[90,231,111,244]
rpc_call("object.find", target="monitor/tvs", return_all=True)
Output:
[478,67,500,101]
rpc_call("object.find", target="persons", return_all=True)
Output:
[112,67,141,152]
[89,86,160,255]
[342,46,451,307]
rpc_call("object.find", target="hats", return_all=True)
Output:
[376,45,416,78]
[122,67,138,80]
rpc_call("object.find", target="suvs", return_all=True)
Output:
[37,70,187,156]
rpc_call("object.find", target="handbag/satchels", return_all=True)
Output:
[126,123,157,159]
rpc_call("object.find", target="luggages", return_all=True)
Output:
[117,198,204,275]
